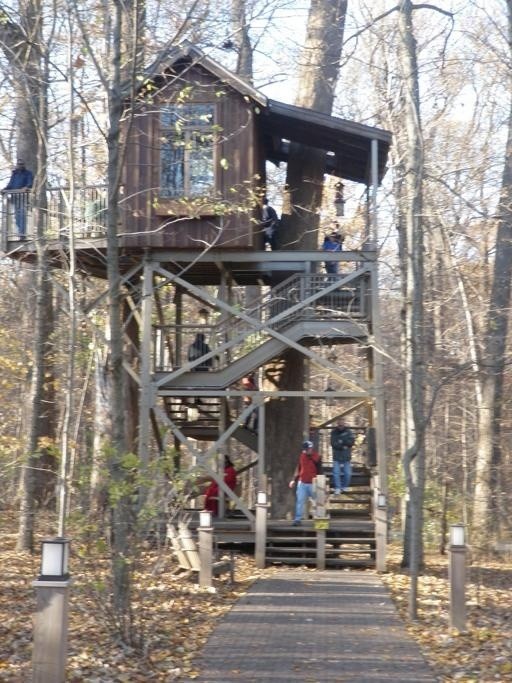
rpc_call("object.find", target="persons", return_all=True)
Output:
[241,369,261,438]
[205,452,237,516]
[328,417,355,497]
[286,440,325,529]
[263,197,281,253]
[187,332,220,404]
[0,156,34,241]
[319,231,344,283]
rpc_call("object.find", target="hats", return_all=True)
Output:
[302,440,314,449]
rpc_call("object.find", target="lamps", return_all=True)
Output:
[198,509,213,529]
[450,522,468,548]
[37,536,71,582]
[378,493,387,507]
[257,490,268,504]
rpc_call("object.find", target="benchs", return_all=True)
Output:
[167,521,237,587]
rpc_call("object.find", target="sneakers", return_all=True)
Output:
[335,487,351,496]
